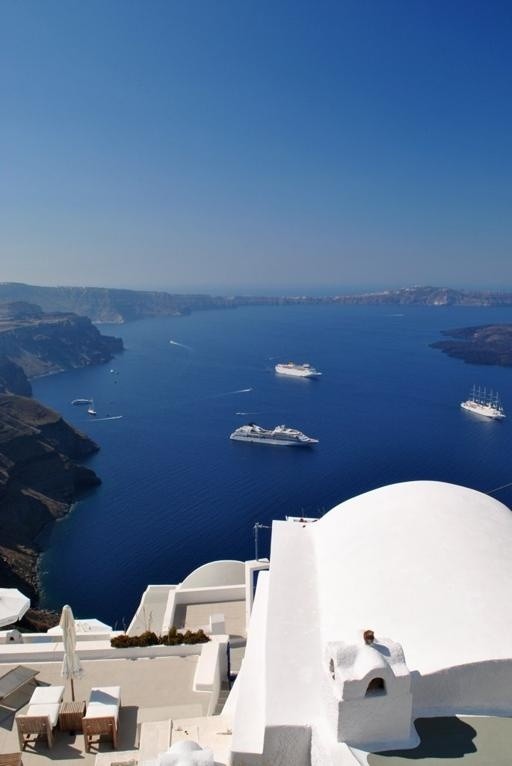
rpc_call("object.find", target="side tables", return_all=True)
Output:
[59,701,85,733]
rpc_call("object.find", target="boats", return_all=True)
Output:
[62,397,125,422]
[461,384,508,420]
[275,360,323,380]
[228,422,321,451]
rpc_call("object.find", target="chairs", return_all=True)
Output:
[14,686,64,751]
[81,686,122,753]
[0,665,41,725]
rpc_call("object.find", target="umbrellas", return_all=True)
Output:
[57,603,85,702]
[0,586,32,629]
